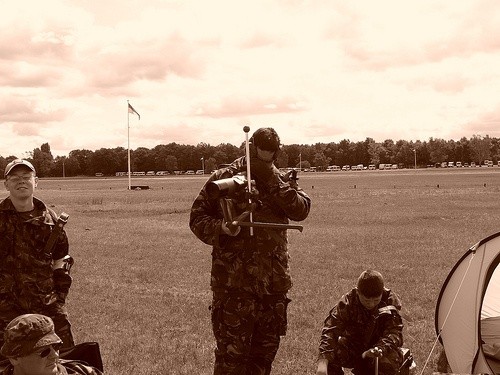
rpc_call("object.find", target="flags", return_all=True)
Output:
[127,103,141,120]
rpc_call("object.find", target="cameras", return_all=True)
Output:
[205,173,259,201]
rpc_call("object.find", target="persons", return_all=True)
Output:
[189,128,312,375]
[0,313,106,375]
[316,269,405,375]
[0,158,76,349]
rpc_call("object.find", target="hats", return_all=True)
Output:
[1,314,63,357]
[4,159,35,178]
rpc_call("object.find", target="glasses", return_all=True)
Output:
[31,342,64,358]
[6,174,35,181]
